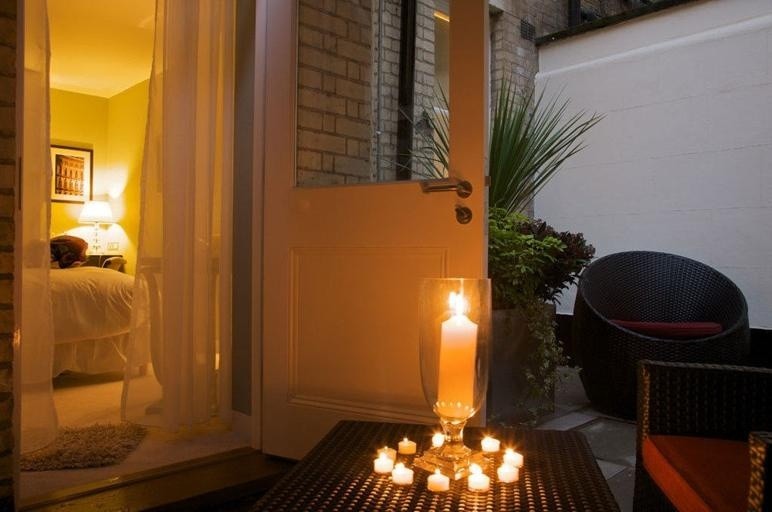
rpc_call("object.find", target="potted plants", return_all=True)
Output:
[490,204,595,427]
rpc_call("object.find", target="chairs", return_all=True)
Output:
[102,257,127,271]
[634,359,772,511]
[573,252,747,421]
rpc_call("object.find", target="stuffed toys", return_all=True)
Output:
[50,234,88,268]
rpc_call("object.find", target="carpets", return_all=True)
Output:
[19,423,147,470]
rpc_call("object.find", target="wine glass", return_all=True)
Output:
[419,276,493,478]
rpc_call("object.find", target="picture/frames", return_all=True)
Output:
[50,145,93,204]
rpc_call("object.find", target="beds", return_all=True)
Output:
[21,266,148,383]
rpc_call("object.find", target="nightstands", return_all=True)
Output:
[86,253,123,267]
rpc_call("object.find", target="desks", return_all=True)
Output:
[251,419,620,512]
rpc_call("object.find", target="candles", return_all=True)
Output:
[373,292,526,493]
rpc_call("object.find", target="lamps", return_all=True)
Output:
[77,200,116,254]
